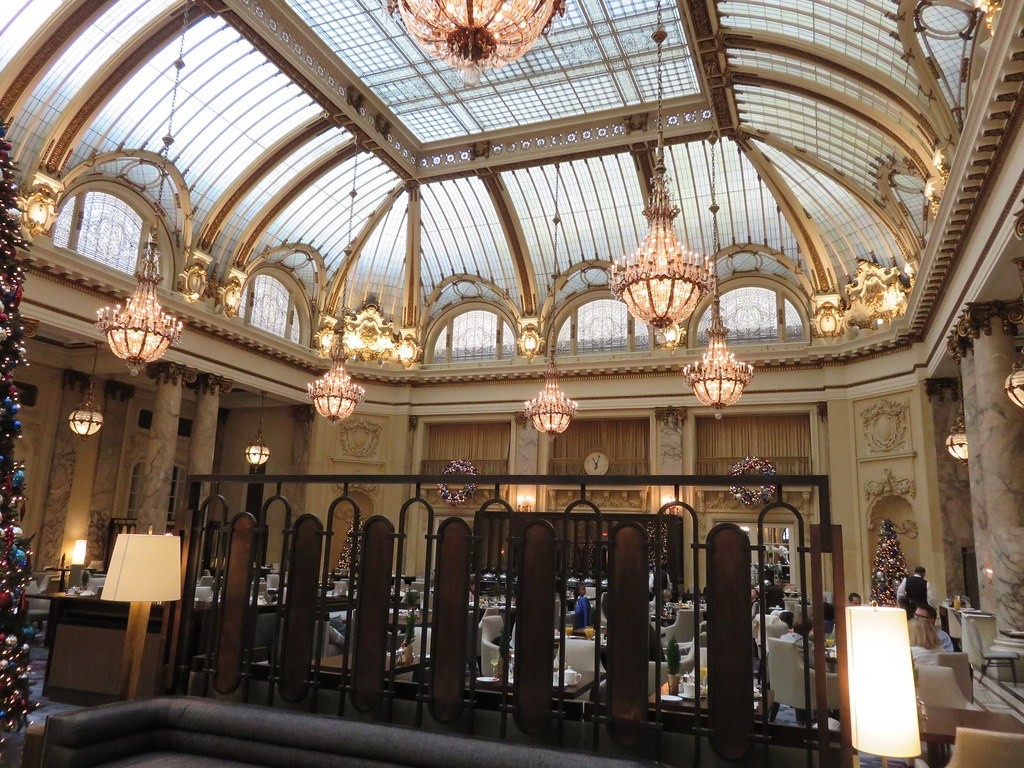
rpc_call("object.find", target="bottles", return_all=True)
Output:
[479,595,506,607]
[953,595,960,611]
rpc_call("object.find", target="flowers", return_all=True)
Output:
[728,452,778,507]
[435,457,478,506]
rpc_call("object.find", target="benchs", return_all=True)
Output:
[40,694,652,768]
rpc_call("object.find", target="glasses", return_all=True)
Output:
[912,612,930,620]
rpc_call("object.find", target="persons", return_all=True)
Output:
[648,568,671,609]
[779,601,836,644]
[575,585,591,628]
[897,566,933,607]
[848,593,861,606]
[897,597,954,667]
[469,581,475,606]
[751,586,761,614]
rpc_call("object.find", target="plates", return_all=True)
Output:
[660,695,683,701]
[678,693,696,699]
[553,678,568,687]
[66,594,75,596]
[476,676,500,682]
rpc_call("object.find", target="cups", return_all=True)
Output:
[564,666,582,685]
[566,624,573,637]
[683,683,696,698]
[585,625,594,639]
[67,589,75,595]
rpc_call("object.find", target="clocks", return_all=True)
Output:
[581,447,612,478]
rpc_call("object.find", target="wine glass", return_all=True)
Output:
[490,654,499,677]
[824,633,834,652]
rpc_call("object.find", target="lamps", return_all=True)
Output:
[607,0,716,331]
[100,532,183,698]
[944,363,970,466]
[1003,257,1024,411]
[524,161,578,439]
[845,604,922,768]
[387,0,568,93]
[683,108,754,422]
[67,343,104,441]
[244,392,271,473]
[305,137,365,424]
[94,0,190,377]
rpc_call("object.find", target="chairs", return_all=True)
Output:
[20,568,1023,768]
